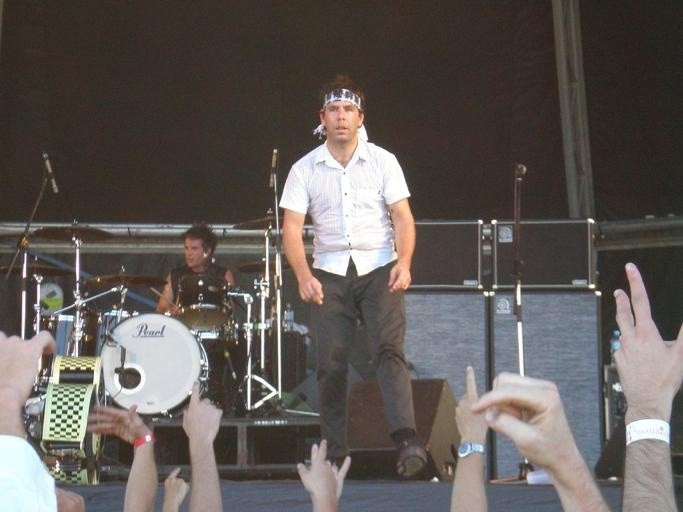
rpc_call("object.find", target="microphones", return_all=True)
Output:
[43,153,59,193]
[269,145,278,188]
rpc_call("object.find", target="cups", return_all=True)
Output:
[525,468,551,485]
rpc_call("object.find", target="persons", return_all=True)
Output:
[156,224,236,322]
[612,263,683,512]
[279,74,427,480]
[297,439,351,511]
[0,330,227,512]
[451,364,608,512]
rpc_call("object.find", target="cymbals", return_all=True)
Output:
[82,274,168,290]
[34,227,115,242]
[0,264,76,277]
[237,260,290,273]
[233,216,313,230]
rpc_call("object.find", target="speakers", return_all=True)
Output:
[407,219,484,291]
[347,378,461,481]
[491,218,595,291]
[595,217,683,458]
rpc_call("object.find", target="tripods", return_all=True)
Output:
[230,304,278,418]
[250,188,319,417]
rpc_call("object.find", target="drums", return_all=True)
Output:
[178,273,232,331]
[40,314,94,385]
[97,310,211,418]
[220,319,245,345]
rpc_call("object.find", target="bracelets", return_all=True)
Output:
[626,420,670,447]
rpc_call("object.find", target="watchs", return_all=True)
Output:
[458,442,485,459]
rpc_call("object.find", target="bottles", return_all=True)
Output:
[283,302,293,332]
[610,331,620,365]
